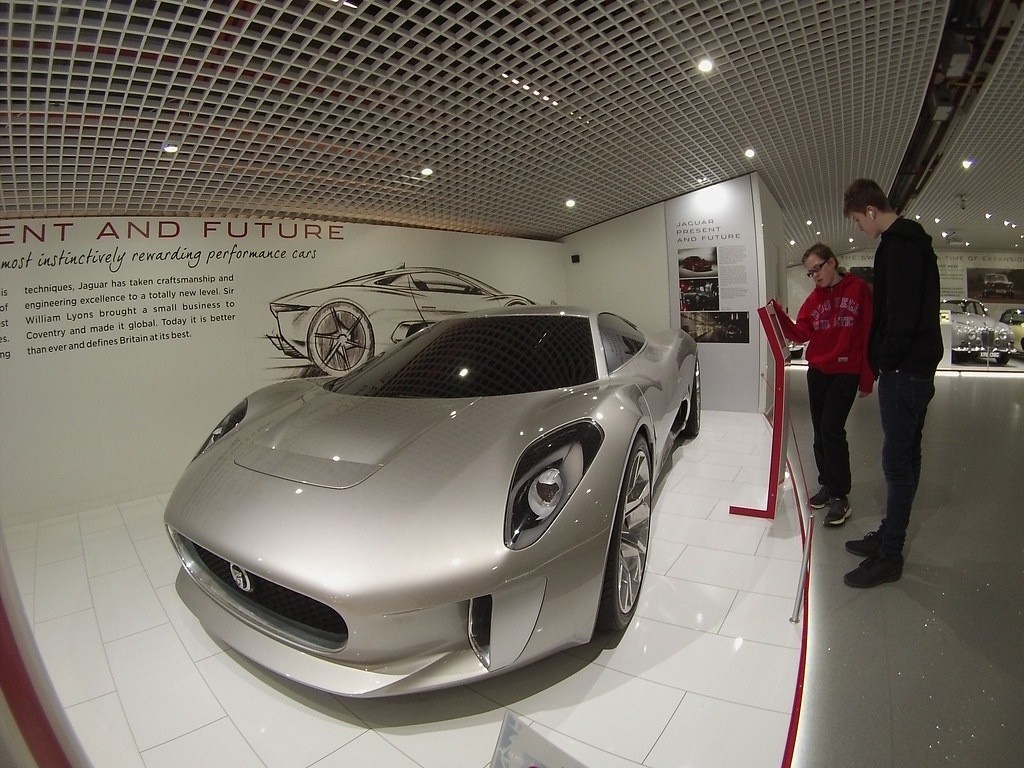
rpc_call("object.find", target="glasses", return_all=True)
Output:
[806,261,826,277]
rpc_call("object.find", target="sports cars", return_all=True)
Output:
[162,304,702,699]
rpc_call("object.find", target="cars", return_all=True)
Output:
[998,307,1024,354]
[983,273,1016,300]
[939,295,1018,366]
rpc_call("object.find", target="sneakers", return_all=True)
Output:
[809,486,830,509]
[823,496,851,524]
[844,556,903,588]
[845,531,877,556]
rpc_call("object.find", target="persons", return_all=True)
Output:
[765,242,873,527]
[842,178,944,589]
